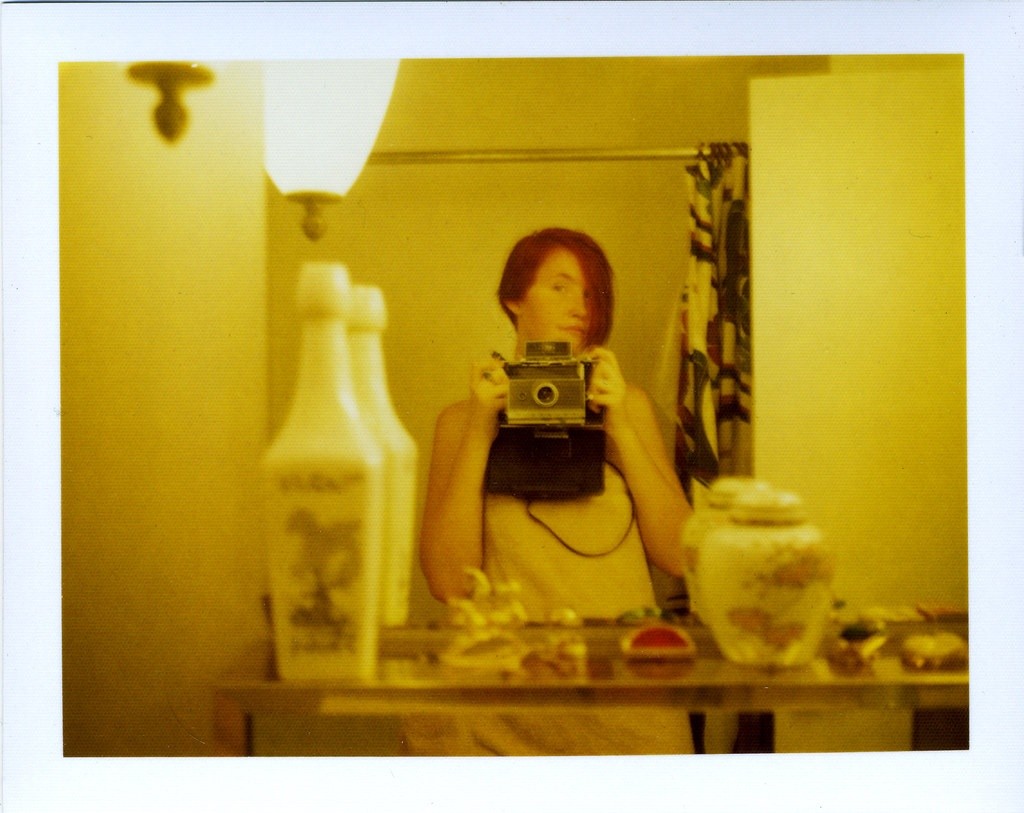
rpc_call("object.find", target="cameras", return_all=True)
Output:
[498,340,608,439]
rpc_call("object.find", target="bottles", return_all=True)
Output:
[264,259,382,683]
[343,283,418,628]
[695,488,841,669]
[679,480,773,616]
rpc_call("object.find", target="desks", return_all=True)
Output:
[210,618,969,755]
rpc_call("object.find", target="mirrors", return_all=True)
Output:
[265,58,762,633]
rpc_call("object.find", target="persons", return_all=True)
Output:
[417,227,695,754]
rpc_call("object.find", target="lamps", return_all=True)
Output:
[264,59,401,240]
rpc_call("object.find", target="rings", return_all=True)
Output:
[483,372,497,386]
